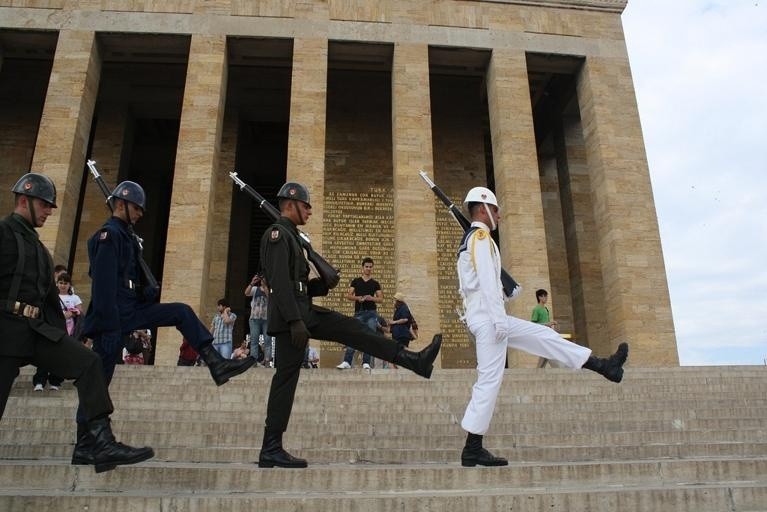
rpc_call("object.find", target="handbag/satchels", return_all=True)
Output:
[408,315,419,339]
[73,310,85,337]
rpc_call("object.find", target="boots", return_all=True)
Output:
[397,333,443,379]
[72,416,155,474]
[259,426,309,469]
[590,342,629,383]
[199,344,256,387]
[461,432,508,467]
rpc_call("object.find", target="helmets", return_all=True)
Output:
[12,173,59,208]
[464,187,500,212]
[111,179,145,209]
[278,181,311,206]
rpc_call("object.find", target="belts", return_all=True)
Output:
[12,301,42,319]
[269,281,309,296]
[121,278,143,293]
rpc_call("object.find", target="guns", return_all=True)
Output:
[87,161,159,291]
[230,173,340,282]
[419,170,520,299]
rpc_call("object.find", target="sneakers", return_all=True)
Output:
[362,363,371,369]
[34,384,44,392]
[49,385,59,390]
[337,361,352,370]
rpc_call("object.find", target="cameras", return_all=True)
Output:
[258,271,264,280]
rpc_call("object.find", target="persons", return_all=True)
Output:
[0,173,154,473]
[71,179,257,465]
[258,183,443,469]
[531,289,559,368]
[457,187,628,466]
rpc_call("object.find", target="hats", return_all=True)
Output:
[393,292,405,303]
